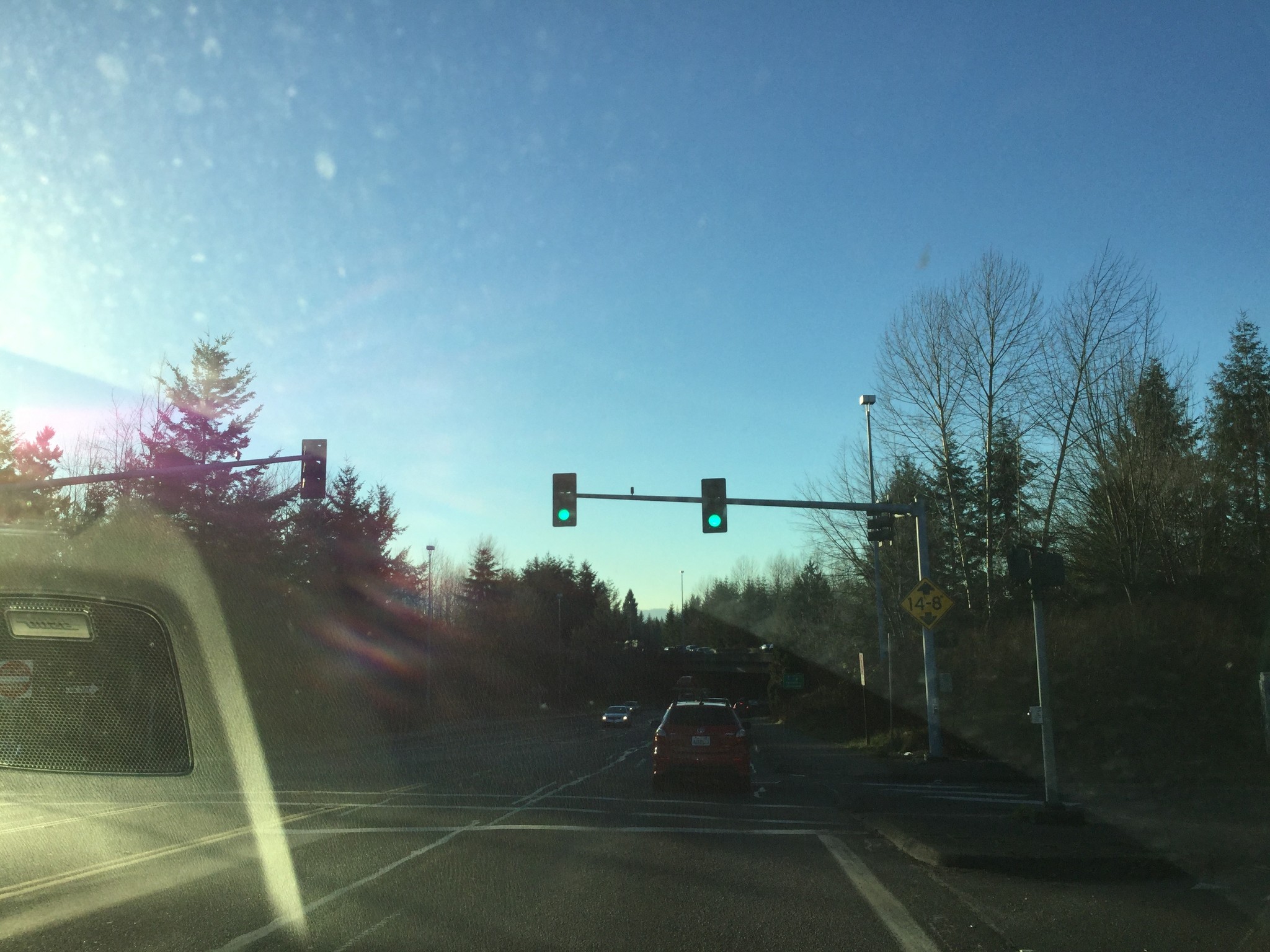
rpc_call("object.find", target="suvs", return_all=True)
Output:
[700,697,730,704]
[651,699,751,789]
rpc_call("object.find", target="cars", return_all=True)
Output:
[602,706,631,729]
[664,645,724,654]
[733,701,749,718]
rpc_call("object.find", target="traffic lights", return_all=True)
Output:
[553,472,577,527]
[701,478,727,533]
[866,493,894,542]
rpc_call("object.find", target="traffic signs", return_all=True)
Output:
[60,683,100,696]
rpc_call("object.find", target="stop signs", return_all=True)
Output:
[0,659,34,698]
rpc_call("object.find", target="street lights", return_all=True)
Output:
[681,570,683,648]
[859,394,887,664]
[426,545,435,733]
[556,593,563,716]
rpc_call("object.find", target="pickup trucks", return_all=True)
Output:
[622,701,642,715]
